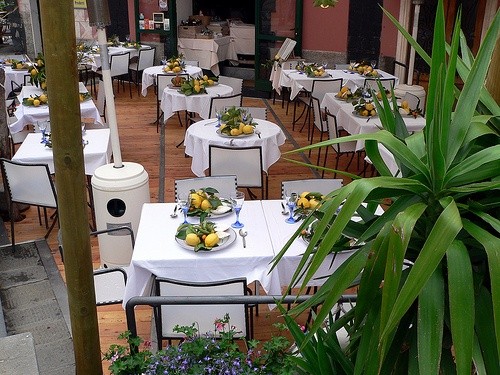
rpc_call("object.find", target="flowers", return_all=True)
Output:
[105,309,296,375]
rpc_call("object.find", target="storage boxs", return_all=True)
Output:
[178,14,229,39]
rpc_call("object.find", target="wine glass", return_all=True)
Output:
[230,191,245,228]
[161,56,167,68]
[177,193,191,225]
[215,107,249,128]
[285,189,298,224]
[298,60,377,74]
[37,118,88,149]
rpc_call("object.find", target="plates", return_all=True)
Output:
[178,198,235,219]
[335,94,418,118]
[297,70,384,78]
[216,117,254,137]
[302,218,365,253]
[162,63,218,94]
[28,92,92,106]
[175,222,236,252]
[295,198,363,216]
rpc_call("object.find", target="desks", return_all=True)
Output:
[141,64,202,98]
[229,24,255,59]
[8,81,105,136]
[344,69,399,92]
[159,83,234,148]
[318,89,396,159]
[177,36,240,76]
[185,117,287,200]
[335,102,427,172]
[279,69,348,131]
[9,128,113,229]
[261,198,387,336]
[4,62,39,98]
[270,57,314,108]
[76,41,151,99]
[122,200,282,354]
[363,141,404,178]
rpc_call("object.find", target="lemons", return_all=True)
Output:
[338,87,410,116]
[187,191,214,210]
[348,65,378,76]
[163,58,214,93]
[7,58,83,107]
[185,224,219,247]
[79,43,139,52]
[295,192,327,211]
[314,70,324,76]
[220,121,253,135]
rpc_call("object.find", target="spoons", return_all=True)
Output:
[255,130,261,138]
[281,202,288,215]
[239,229,248,248]
[171,205,177,218]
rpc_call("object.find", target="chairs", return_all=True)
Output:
[0,46,422,349]
